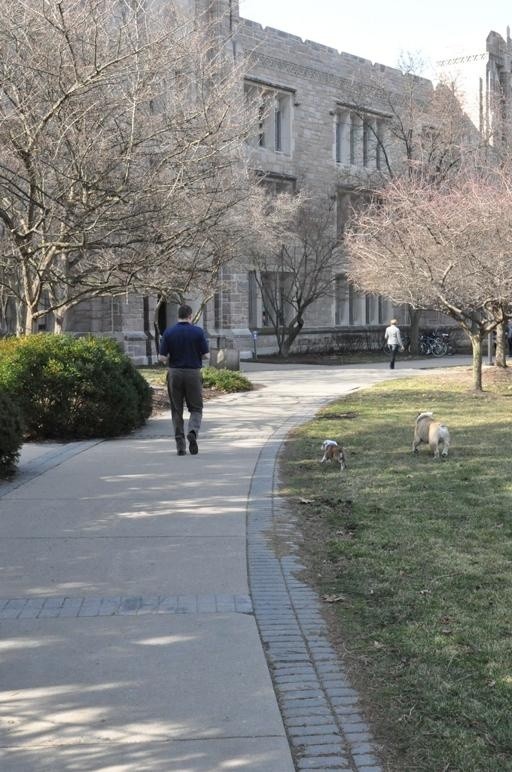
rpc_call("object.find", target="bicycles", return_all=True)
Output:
[383,330,455,357]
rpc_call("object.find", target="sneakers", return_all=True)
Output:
[187,432,198,456]
[177,452,186,456]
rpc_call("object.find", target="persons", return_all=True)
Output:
[384,318,404,368]
[505,320,511,357]
[157,304,211,457]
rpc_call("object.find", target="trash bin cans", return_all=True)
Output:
[210,348,240,371]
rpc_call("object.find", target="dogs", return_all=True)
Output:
[412,410,451,460]
[319,439,350,472]
